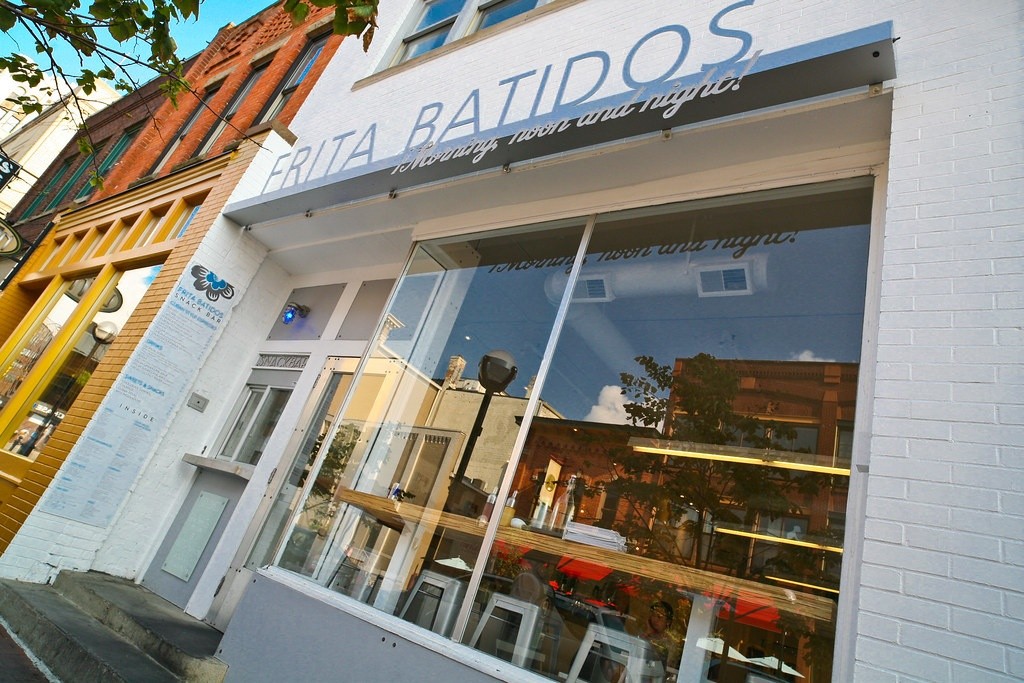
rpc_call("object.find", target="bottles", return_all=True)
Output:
[499,490,519,528]
[480,487,499,522]
[548,474,578,533]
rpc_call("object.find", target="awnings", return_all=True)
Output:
[492,538,788,634]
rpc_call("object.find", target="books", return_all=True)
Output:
[522,525,563,539]
[562,522,628,551]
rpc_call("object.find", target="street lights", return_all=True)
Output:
[394,348,519,616]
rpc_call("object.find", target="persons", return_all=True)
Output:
[612,600,682,683]
[566,469,587,521]
[477,555,556,656]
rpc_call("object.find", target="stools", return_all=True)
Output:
[399,570,465,638]
[565,624,662,683]
[469,593,545,670]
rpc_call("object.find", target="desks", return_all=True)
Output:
[340,490,838,683]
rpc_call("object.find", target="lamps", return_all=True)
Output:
[281,301,310,325]
